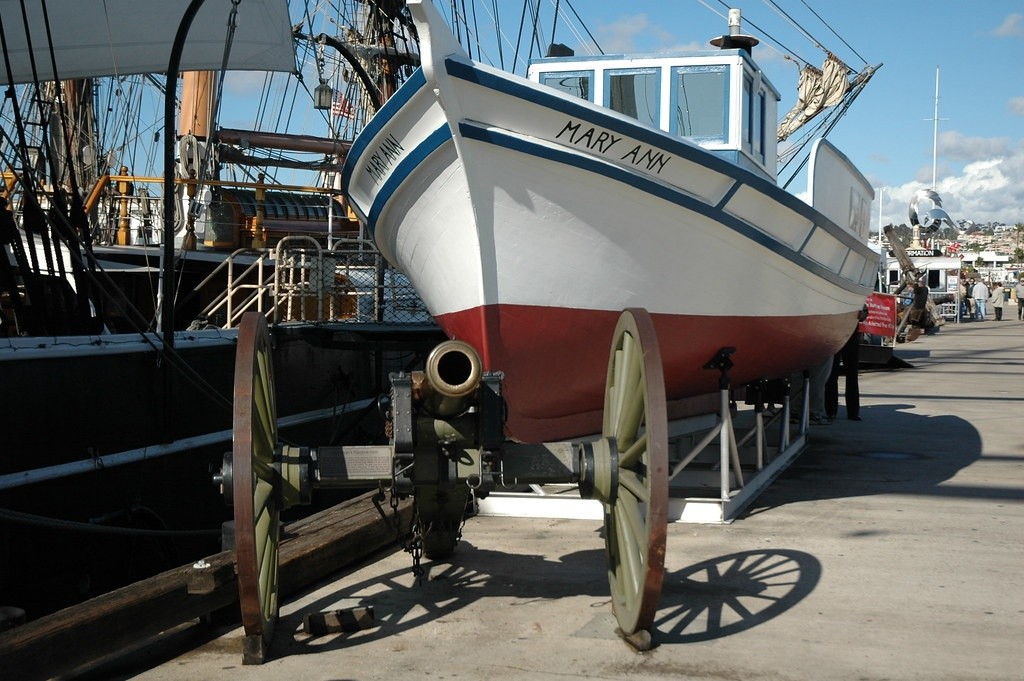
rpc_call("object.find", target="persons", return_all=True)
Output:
[1015,278,1024,320]
[973,281,988,319]
[992,282,1004,321]
[967,278,975,297]
[957,282,968,319]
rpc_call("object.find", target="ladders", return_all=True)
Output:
[4,89,57,215]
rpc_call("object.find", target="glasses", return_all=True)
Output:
[969,281,974,282]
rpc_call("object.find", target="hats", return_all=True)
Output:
[1020,278,1024,281]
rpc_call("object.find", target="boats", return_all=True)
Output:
[339,6,888,447]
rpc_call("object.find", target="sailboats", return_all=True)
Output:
[0,0,883,624]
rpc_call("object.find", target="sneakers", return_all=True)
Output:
[789,412,833,424]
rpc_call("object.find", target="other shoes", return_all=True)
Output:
[998,318,1000,320]
[992,318,997,321]
[830,414,836,418]
[755,403,780,416]
[847,413,861,421]
